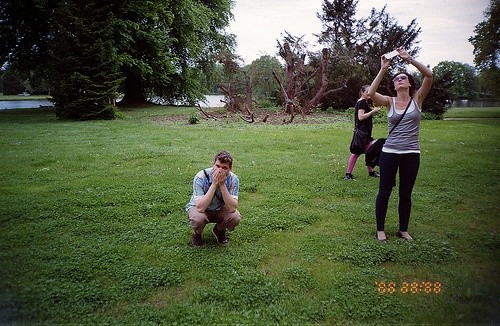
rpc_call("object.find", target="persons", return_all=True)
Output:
[185,150,241,247]
[344,85,381,181]
[368,48,434,243]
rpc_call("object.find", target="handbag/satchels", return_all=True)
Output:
[349,129,371,154]
[365,138,386,169]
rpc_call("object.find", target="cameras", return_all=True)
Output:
[384,50,400,60]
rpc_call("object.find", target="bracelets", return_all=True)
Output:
[407,57,415,64]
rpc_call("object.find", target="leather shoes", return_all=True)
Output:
[376,232,388,243]
[397,231,413,240]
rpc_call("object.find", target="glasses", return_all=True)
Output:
[392,76,409,82]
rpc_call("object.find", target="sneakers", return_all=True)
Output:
[344,174,357,181]
[212,224,228,245]
[369,171,380,177]
[193,233,203,245]
